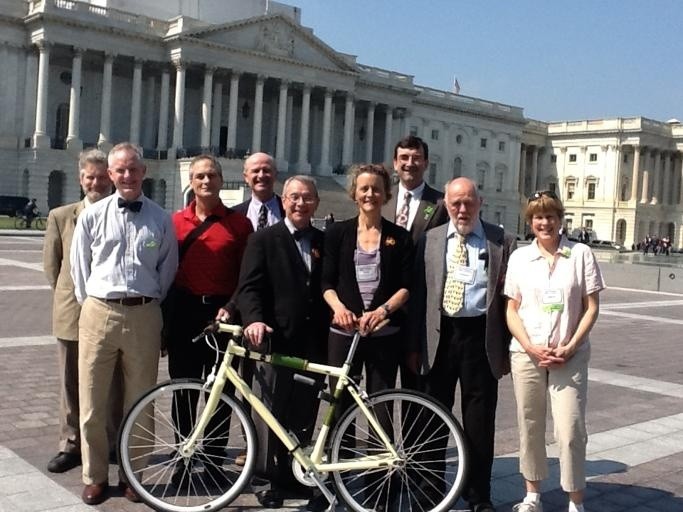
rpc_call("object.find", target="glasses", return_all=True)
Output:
[526,190,562,207]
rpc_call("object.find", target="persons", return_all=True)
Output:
[68,139,178,504]
[417,176,512,511]
[24,198,40,230]
[577,227,589,244]
[227,151,288,488]
[324,213,334,227]
[317,164,414,509]
[158,153,254,484]
[42,149,127,472]
[641,233,672,258]
[238,176,329,506]
[504,194,603,511]
[381,135,449,486]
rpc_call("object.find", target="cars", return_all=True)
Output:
[592,240,626,251]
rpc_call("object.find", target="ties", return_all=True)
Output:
[442,233,467,317]
[394,192,411,229]
[257,205,268,231]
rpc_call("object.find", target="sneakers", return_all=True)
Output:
[512,496,543,512]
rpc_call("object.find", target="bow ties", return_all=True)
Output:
[118,198,142,213]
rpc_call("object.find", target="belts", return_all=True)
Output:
[106,295,153,307]
[196,295,224,304]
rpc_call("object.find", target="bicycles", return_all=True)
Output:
[117,312,468,511]
[12,211,49,231]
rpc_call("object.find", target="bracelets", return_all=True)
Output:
[380,305,391,315]
